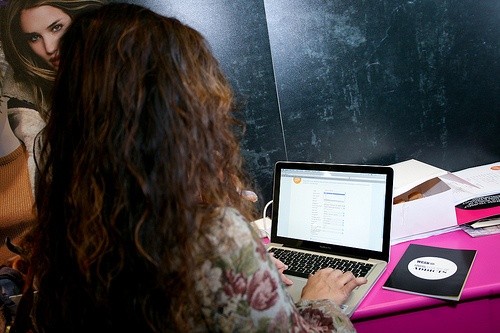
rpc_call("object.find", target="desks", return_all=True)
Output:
[248,161,500,333]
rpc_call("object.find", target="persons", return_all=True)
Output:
[10,4,366,333]
[0,0,121,200]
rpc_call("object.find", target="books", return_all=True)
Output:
[382,243,478,301]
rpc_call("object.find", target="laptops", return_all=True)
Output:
[263,162,394,323]
